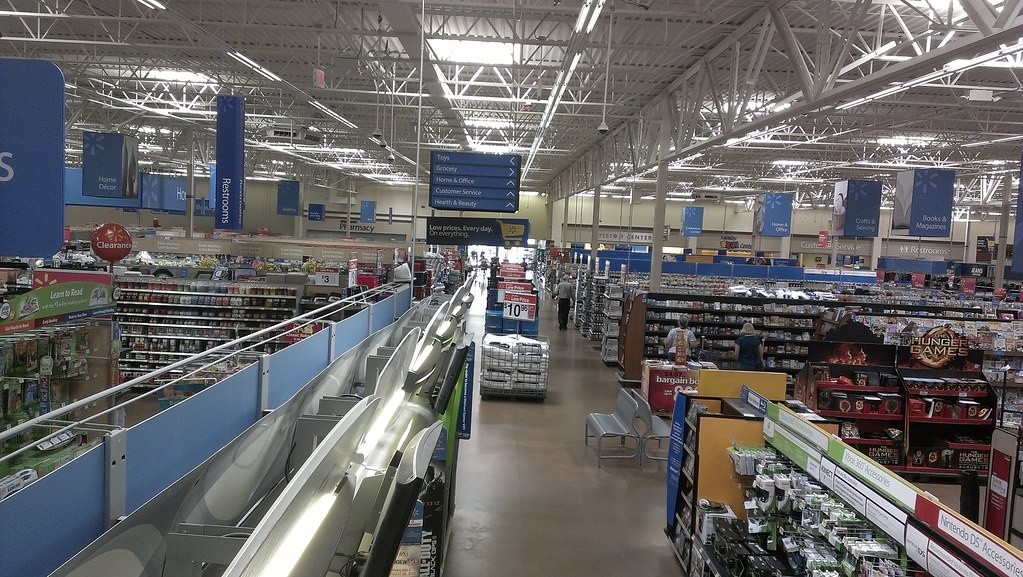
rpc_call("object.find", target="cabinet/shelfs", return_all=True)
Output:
[0,223,1023,576]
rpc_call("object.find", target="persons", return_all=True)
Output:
[555,274,576,331]
[453,258,463,272]
[520,257,528,272]
[732,320,765,370]
[665,316,701,363]
[479,255,489,273]
[465,256,473,281]
